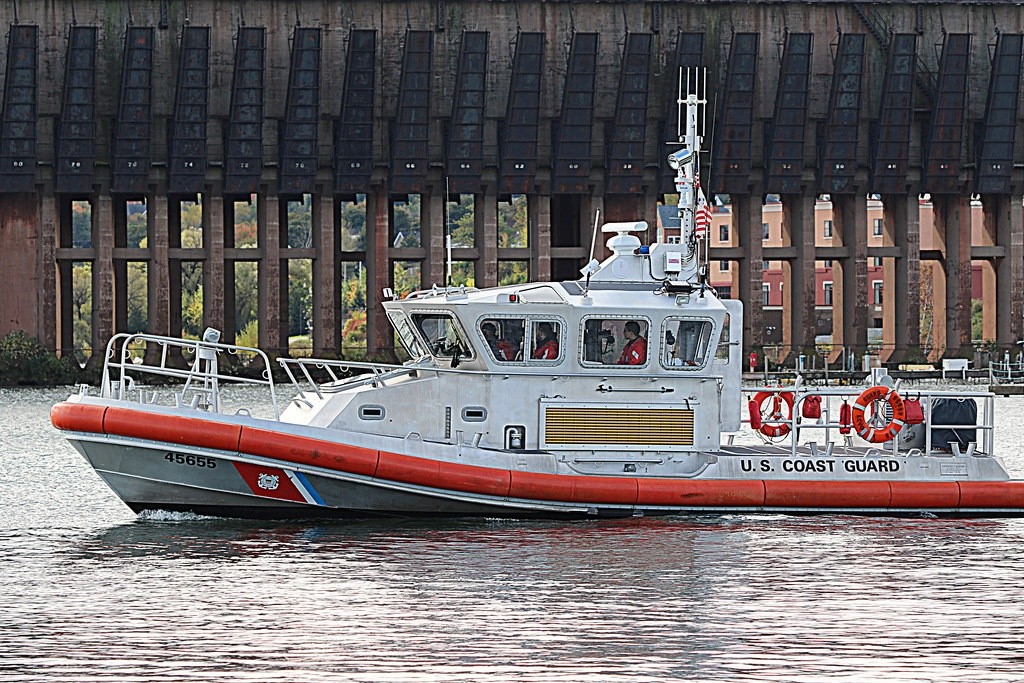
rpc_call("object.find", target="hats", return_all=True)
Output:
[481,323,496,337]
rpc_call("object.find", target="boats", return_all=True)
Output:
[50,67,1023,518]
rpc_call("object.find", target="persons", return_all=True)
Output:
[533,322,558,358]
[482,323,516,361]
[617,321,647,365]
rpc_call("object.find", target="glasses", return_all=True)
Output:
[624,328,627,332]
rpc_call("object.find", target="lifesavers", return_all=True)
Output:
[852,385,904,444]
[750,384,799,438]
